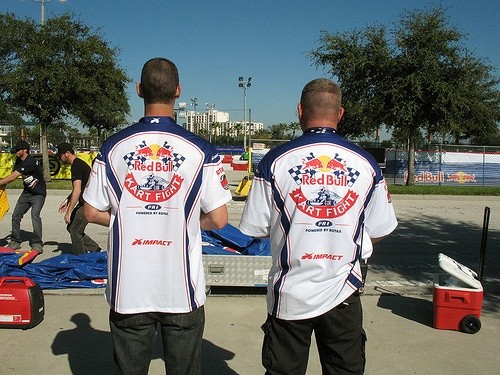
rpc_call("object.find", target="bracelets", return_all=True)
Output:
[65,198,69,203]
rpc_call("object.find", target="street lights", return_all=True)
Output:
[238,76,253,151]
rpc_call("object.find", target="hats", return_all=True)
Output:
[55,143,73,156]
[11,141,30,153]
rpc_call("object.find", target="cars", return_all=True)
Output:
[239,153,249,161]
[0,146,58,154]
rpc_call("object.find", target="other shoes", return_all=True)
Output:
[31,243,43,253]
[7,241,21,250]
[90,247,102,253]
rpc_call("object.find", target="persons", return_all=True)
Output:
[0,140,47,252]
[83,58,232,375]
[54,142,101,255]
[238,77,398,375]
